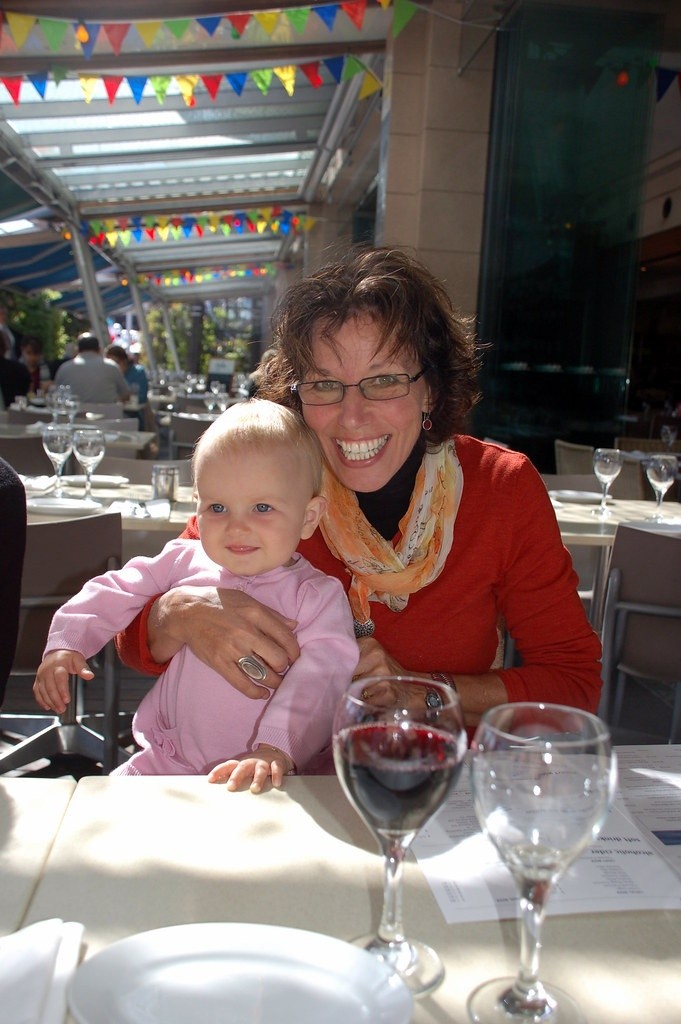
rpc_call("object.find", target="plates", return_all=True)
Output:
[64,475,129,487]
[27,497,103,516]
[64,922,414,1024]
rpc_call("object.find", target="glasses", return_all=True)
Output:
[290,366,431,406]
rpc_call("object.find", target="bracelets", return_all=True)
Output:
[431,671,456,691]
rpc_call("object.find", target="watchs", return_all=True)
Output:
[425,686,439,721]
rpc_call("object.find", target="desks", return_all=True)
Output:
[1,773,77,939]
[22,475,203,773]
[65,425,158,459]
[15,740,681,1023]
[534,497,678,636]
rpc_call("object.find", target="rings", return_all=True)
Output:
[362,689,369,699]
[238,656,266,680]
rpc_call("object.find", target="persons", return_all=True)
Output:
[33,398,359,793]
[104,346,148,402]
[114,246,604,775]
[55,331,130,403]
[18,336,57,393]
[0,323,31,407]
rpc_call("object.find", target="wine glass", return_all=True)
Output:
[42,424,72,499]
[591,448,624,521]
[72,429,106,502]
[216,392,230,414]
[44,385,80,424]
[331,676,468,1002]
[645,455,677,522]
[465,700,620,1024]
[203,393,216,417]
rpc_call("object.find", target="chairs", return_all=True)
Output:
[0,392,681,772]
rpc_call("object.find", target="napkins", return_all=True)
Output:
[0,917,86,1024]
